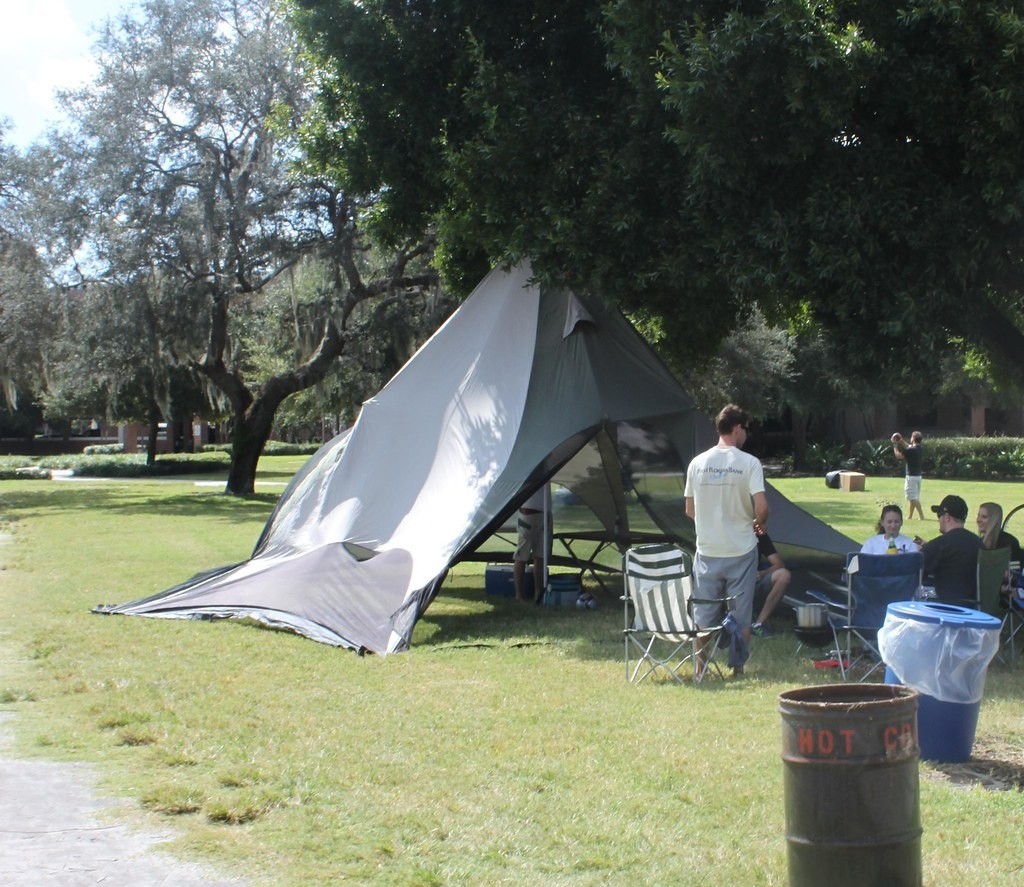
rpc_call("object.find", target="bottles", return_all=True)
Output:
[887,533,898,555]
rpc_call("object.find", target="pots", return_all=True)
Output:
[792,603,830,627]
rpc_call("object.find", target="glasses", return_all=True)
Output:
[937,511,949,518]
[742,424,752,434]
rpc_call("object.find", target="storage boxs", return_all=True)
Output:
[839,472,865,491]
[484,564,549,600]
[544,573,582,607]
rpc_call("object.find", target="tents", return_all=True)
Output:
[93,248,870,655]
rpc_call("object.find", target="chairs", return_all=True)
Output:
[620,542,745,688]
[996,562,1024,670]
[797,553,925,682]
[928,544,1015,674]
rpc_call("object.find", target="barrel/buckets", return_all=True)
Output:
[779,683,925,887]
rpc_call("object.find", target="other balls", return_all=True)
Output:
[891,432,902,443]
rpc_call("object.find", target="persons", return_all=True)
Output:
[514,484,553,603]
[891,431,924,522]
[912,495,987,606]
[999,572,1024,613]
[754,533,790,636]
[860,505,920,555]
[977,502,1019,562]
[684,404,768,684]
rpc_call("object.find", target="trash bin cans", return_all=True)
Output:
[876,601,1002,763]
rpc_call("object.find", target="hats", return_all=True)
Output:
[931,495,968,520]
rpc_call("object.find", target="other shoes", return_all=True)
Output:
[750,623,774,640]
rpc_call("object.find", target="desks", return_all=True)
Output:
[552,531,672,596]
